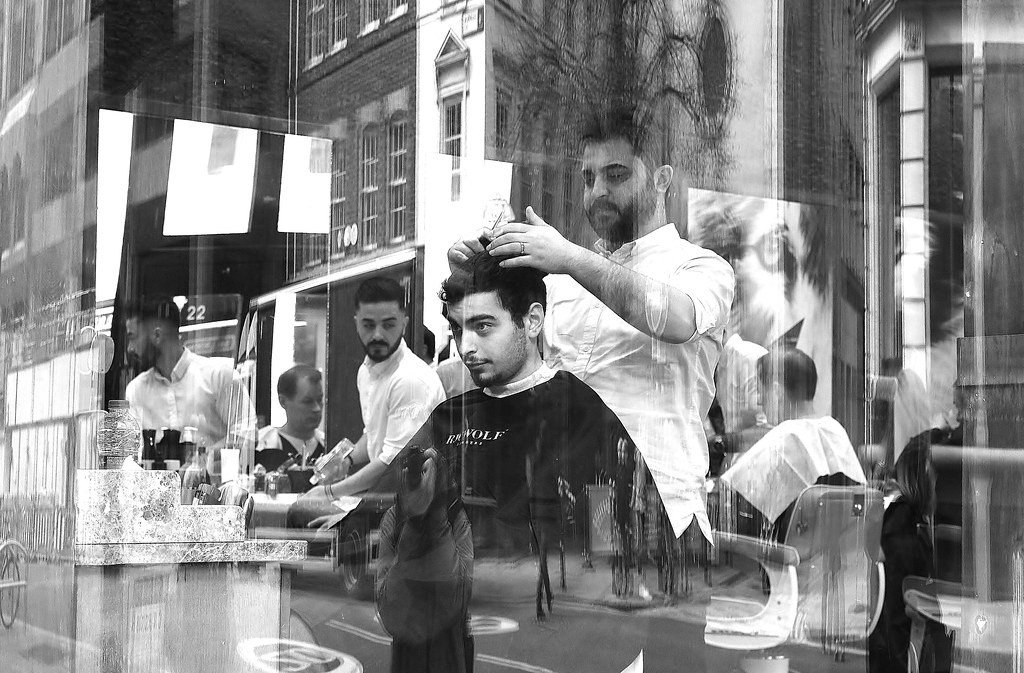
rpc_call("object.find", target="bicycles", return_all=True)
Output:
[0,540,21,629]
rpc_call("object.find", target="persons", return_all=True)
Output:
[249,362,330,493]
[297,238,687,602]
[118,293,260,487]
[374,445,478,671]
[709,335,768,457]
[446,115,734,550]
[722,344,869,594]
[864,326,964,673]
[286,277,480,534]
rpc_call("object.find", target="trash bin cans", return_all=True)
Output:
[232,637,365,672]
[738,654,789,673]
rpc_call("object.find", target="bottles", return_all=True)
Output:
[96,400,141,457]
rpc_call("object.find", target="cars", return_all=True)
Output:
[244,456,404,599]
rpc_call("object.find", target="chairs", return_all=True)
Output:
[704,485,887,672]
[899,445,1024,673]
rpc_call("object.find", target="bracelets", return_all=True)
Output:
[325,483,337,502]
[347,455,355,467]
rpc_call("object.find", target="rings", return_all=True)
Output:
[520,242,525,254]
